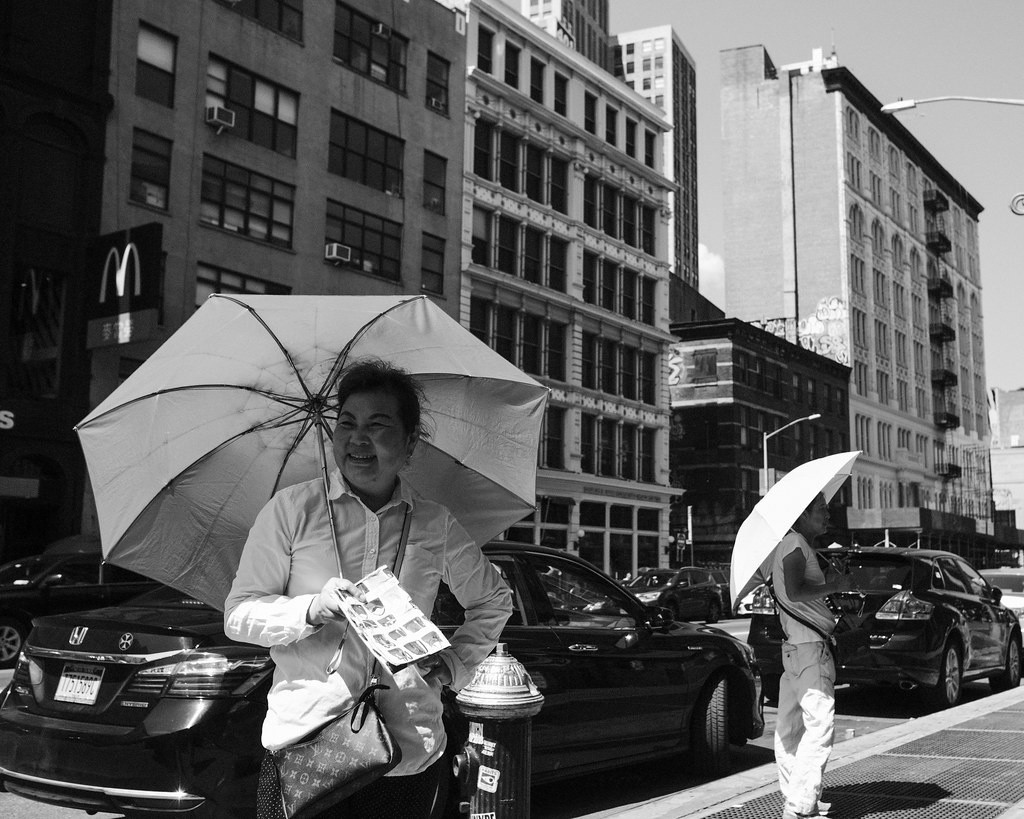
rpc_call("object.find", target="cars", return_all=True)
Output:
[705,569,749,618]
[0,548,162,670]
[0,533,766,819]
[746,542,1024,712]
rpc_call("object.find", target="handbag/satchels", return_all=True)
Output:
[828,616,858,669]
[272,698,402,819]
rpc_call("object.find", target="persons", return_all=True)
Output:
[772,492,858,819]
[225,349,512,819]
[646,575,660,588]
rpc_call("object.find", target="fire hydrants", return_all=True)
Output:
[453,643,546,819]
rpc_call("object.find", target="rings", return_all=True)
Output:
[432,656,438,666]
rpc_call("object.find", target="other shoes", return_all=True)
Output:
[781,810,830,819]
[816,799,831,815]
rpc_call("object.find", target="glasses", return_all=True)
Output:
[807,504,830,513]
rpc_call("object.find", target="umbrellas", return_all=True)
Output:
[71,292,555,677]
[730,450,866,619]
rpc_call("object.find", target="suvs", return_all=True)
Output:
[581,565,725,624]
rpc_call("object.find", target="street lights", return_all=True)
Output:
[764,414,822,492]
